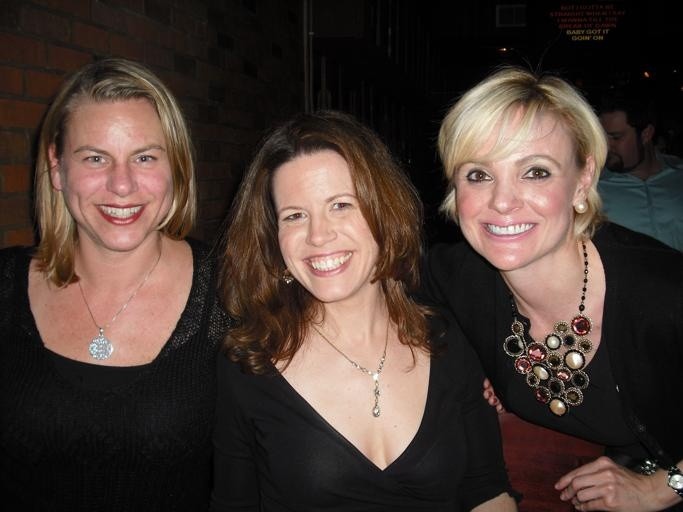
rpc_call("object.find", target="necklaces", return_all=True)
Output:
[57,237,169,360]
[497,240,596,418]
[303,302,398,421]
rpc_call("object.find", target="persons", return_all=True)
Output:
[586,97,682,251]
[0,53,228,511]
[206,107,522,511]
[399,67,682,512]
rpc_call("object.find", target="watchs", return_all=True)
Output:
[664,461,682,506]
